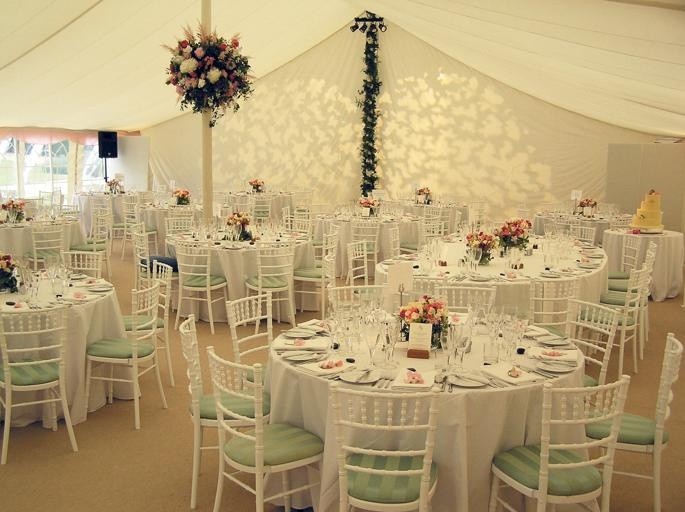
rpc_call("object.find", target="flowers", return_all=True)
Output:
[353,7,383,197]
[248,178,266,185]
[156,20,256,131]
[105,178,120,192]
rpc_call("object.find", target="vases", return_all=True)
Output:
[253,184,262,192]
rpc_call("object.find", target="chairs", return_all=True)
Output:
[2,249,174,465]
[1,188,348,337]
[318,189,683,374]
[179,282,684,511]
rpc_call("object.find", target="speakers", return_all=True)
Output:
[98,131,118,158]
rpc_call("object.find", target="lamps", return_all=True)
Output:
[348,14,388,36]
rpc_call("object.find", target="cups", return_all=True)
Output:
[33,204,60,221]
[184,216,288,243]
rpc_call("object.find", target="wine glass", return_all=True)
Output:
[17,253,73,305]
[323,299,524,373]
[420,220,580,276]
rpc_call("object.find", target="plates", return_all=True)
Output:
[535,338,577,373]
[339,371,380,384]
[70,273,114,292]
[471,275,487,282]
[445,374,489,388]
[277,328,319,365]
[544,238,606,279]
